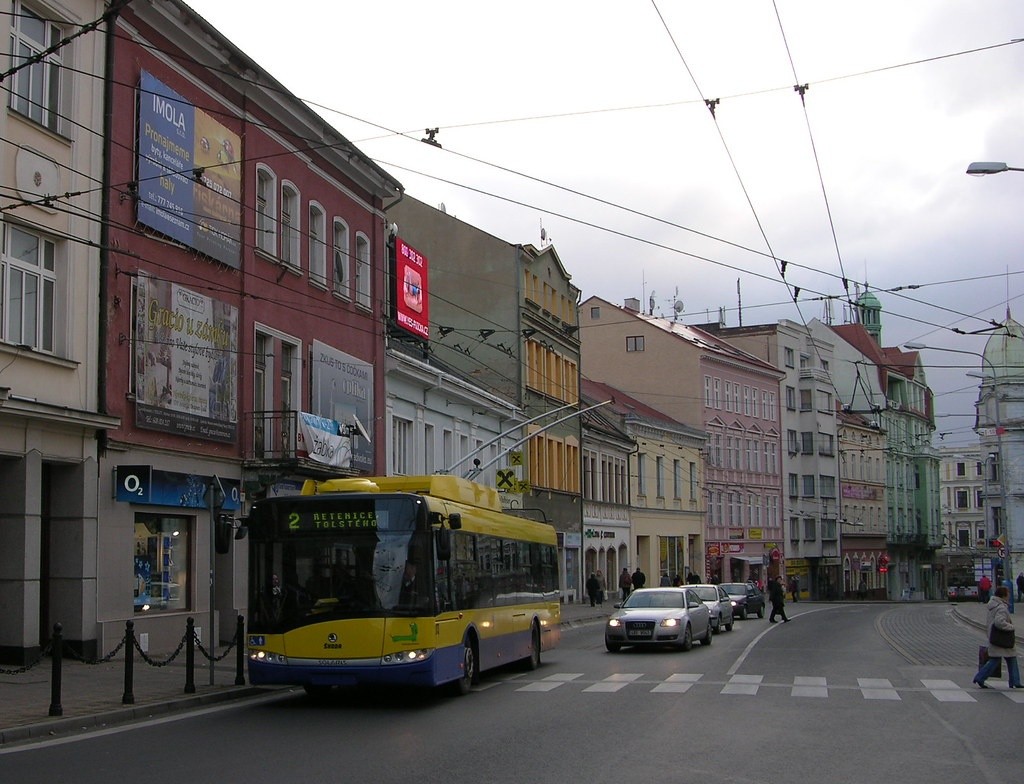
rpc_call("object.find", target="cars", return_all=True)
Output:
[946,577,980,601]
[660,569,672,583]
[133,581,180,612]
[720,583,766,620]
[605,587,712,651]
[680,585,734,635]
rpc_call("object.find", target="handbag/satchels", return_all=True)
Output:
[990,624,1016,649]
[604,589,608,600]
[619,588,623,599]
[978,645,1002,679]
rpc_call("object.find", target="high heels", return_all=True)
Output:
[1008,679,1024,688]
[973,677,987,688]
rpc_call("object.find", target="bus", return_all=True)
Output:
[214,475,560,698]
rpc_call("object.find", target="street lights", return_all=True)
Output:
[984,455,996,554]
[904,341,1015,613]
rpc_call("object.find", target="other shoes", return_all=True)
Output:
[784,619,791,622]
[769,619,778,623]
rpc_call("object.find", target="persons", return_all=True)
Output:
[672,575,682,587]
[632,567,645,591]
[790,576,800,602]
[586,573,600,607]
[979,574,992,604]
[660,573,671,586]
[973,587,1024,688]
[1017,572,1024,603]
[595,570,605,605]
[686,571,701,584]
[399,560,429,604]
[271,573,281,596]
[619,568,633,602]
[710,575,720,585]
[746,578,762,592]
[769,576,791,623]
[859,579,867,602]
